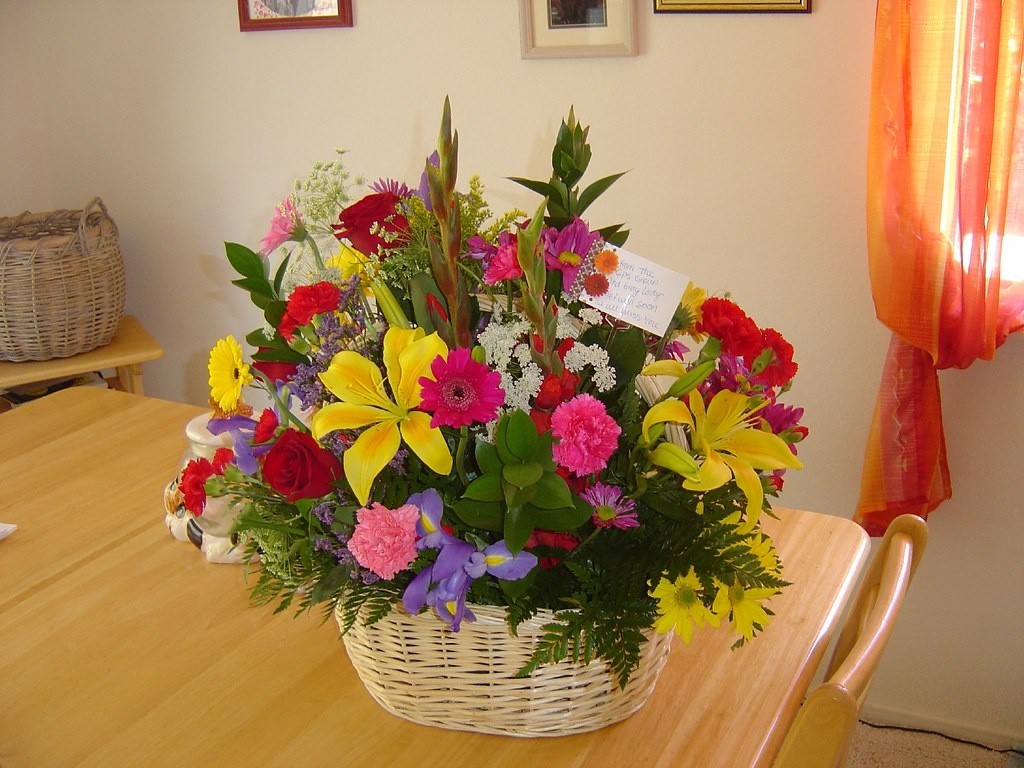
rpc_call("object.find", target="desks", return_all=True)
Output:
[1,384,872,768]
[0,314,165,415]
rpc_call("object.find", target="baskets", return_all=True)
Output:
[0,196,125,364]
[332,292,691,738]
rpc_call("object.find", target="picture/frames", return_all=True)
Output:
[238,0,353,32]
[516,0,638,61]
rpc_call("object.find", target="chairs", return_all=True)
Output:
[770,512,930,768]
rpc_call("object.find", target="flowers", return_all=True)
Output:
[178,96,810,694]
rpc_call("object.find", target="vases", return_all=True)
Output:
[327,581,676,739]
